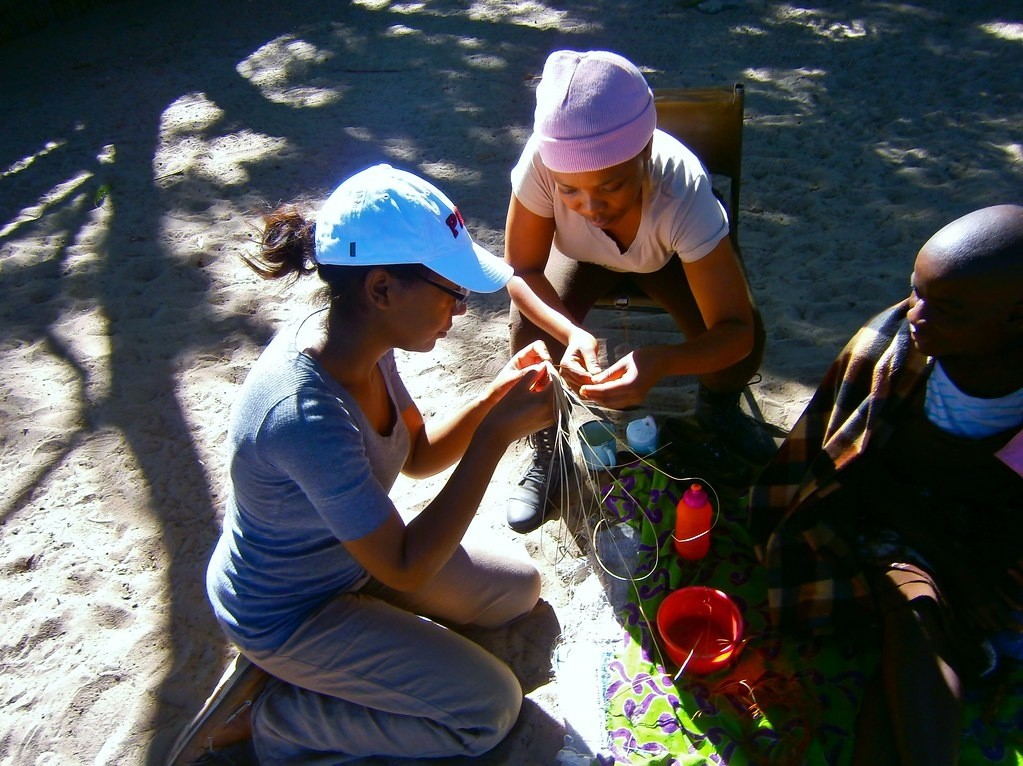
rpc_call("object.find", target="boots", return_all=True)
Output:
[508,400,570,530]
[695,374,782,474]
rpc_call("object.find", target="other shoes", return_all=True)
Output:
[166,652,272,766]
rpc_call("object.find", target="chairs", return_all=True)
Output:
[590,80,769,394]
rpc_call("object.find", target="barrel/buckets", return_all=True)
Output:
[657,586,744,674]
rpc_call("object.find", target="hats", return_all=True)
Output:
[532,51,656,172]
[314,162,514,294]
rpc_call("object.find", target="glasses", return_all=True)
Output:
[408,269,470,311]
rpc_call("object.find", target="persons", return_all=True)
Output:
[503,49,765,530]
[759,203,1023,766]
[164,159,562,766]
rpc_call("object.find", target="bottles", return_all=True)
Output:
[674,483,713,561]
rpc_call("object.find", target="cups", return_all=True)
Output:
[577,420,617,472]
[627,414,658,456]
